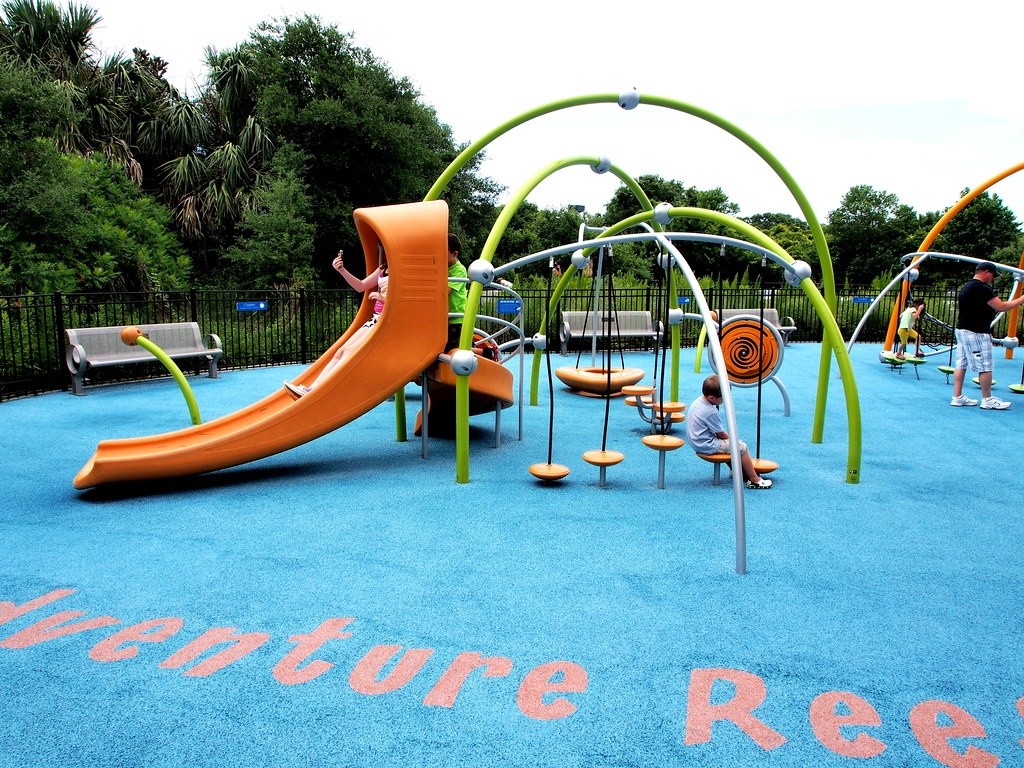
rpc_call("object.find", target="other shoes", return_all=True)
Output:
[896,352,906,360]
[481,346,494,360]
[915,352,924,357]
[283,380,309,401]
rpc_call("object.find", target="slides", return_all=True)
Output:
[71,199,448,492]
[413,348,515,437]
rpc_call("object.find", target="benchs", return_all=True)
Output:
[559,311,664,357]
[64,322,223,396]
[714,308,797,346]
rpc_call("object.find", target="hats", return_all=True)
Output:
[975,262,1000,278]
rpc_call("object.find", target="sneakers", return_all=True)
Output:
[746,477,772,489]
[980,397,1012,410]
[728,469,733,479]
[951,395,978,406]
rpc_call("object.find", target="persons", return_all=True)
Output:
[444,233,494,361]
[950,262,1024,410]
[895,297,926,360]
[283,254,388,402]
[497,258,593,290]
[686,373,771,488]
